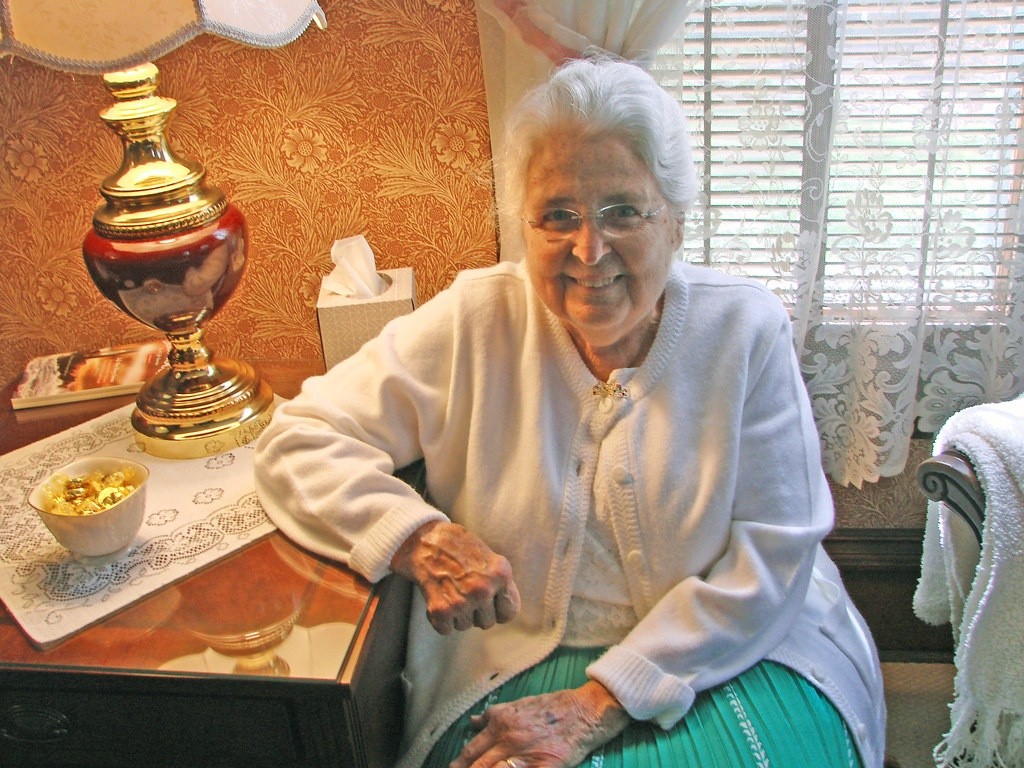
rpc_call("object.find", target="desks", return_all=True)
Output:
[0,356,417,768]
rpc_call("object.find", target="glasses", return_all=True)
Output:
[519,202,668,241]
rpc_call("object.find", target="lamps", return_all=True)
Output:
[0,0,329,461]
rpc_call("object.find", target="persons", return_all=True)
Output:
[255,60,888,768]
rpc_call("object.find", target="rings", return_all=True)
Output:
[505,758,516,768]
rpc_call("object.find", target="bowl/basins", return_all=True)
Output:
[27,457,150,557]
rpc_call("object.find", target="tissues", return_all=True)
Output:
[316,234,419,374]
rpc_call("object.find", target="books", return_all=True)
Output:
[11,340,173,409]
